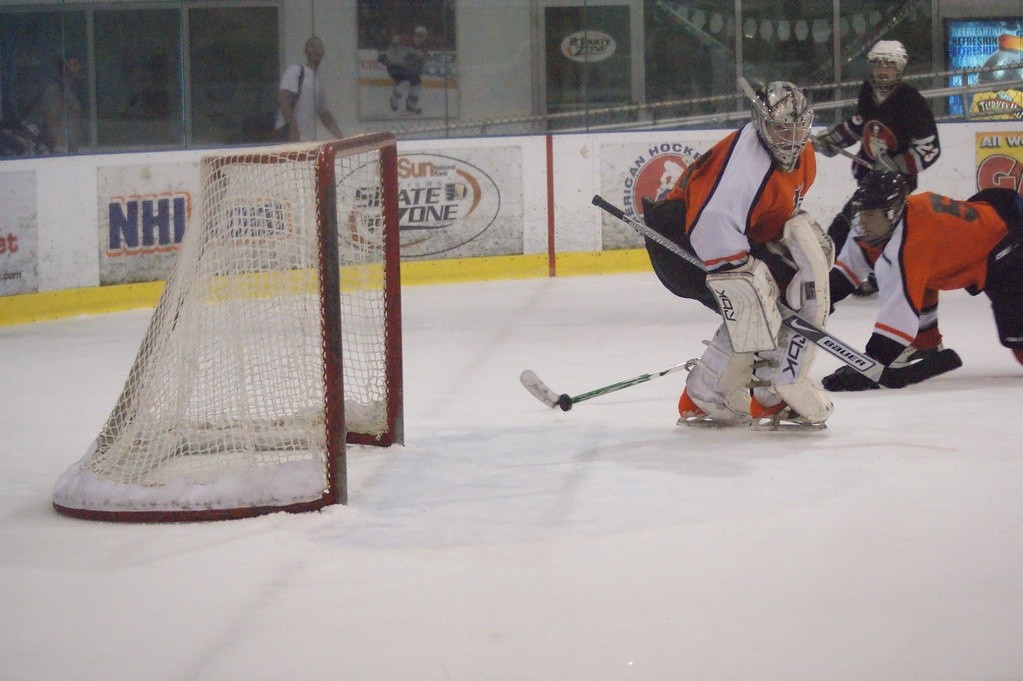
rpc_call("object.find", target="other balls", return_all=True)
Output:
[559,393,572,411]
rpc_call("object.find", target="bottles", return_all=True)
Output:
[969,34,1023,123]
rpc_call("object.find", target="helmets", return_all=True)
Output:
[867,40,908,103]
[752,81,815,174]
[851,170,909,251]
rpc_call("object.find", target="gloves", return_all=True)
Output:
[873,149,918,176]
[821,333,907,391]
[812,124,857,158]
[826,266,855,315]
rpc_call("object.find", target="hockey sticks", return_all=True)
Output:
[590,194,963,390]
[518,357,700,413]
[735,74,875,170]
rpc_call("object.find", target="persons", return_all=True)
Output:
[274,37,345,143]
[30,55,82,152]
[809,40,940,314]
[642,80,835,432]
[381,26,427,113]
[821,170,1023,390]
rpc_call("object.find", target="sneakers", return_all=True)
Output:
[887,317,944,370]
[676,385,753,427]
[751,394,827,432]
[849,273,880,302]
